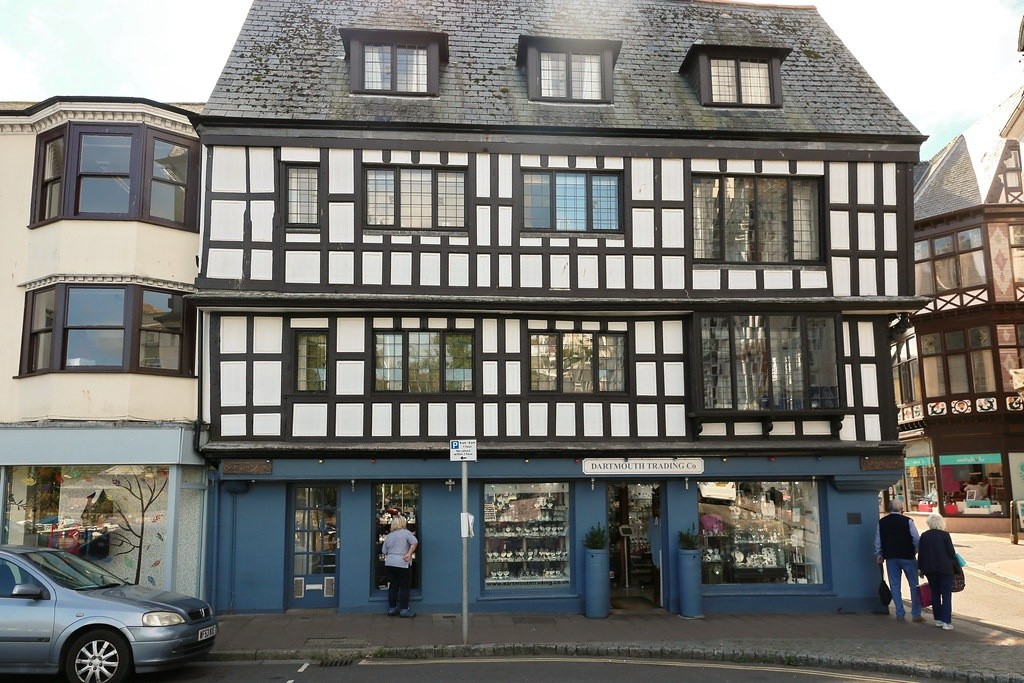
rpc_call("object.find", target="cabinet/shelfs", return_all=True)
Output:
[484,532,569,584]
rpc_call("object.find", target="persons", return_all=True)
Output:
[874,500,926,622]
[963,476,992,499]
[918,513,961,629]
[382,515,419,617]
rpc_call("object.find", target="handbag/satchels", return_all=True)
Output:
[952,567,965,592]
[916,578,932,607]
[879,563,893,606]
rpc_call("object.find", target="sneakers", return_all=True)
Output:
[936,620,943,626]
[943,622,953,630]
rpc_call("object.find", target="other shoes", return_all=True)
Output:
[912,616,926,622]
[897,617,904,621]
[388,606,400,615]
[399,607,415,616]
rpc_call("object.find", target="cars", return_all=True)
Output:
[0,542,219,683]
[0,518,58,549]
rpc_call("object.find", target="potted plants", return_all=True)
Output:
[582,523,612,619]
[677,521,703,616]
